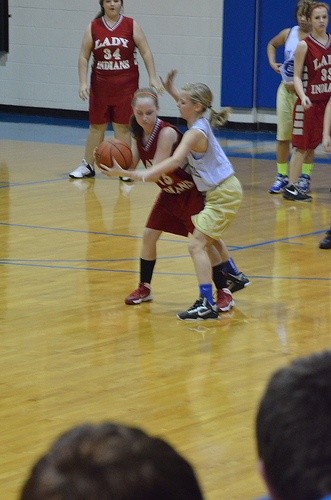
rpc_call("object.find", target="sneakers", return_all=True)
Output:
[283,183,312,202]
[267,173,289,194]
[298,173,311,190]
[212,287,235,312]
[124,281,153,305]
[221,269,251,293]
[175,293,221,321]
[68,158,96,179]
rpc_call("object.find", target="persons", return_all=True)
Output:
[20,421,203,500]
[93,87,235,311]
[267,0,314,194]
[141,69,252,319]
[68,0,164,181]
[283,3,331,201]
[256,350,331,500]
[320,98,331,249]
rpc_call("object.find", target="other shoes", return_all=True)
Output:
[319,228,331,249]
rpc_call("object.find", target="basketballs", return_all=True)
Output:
[95,138,133,173]
